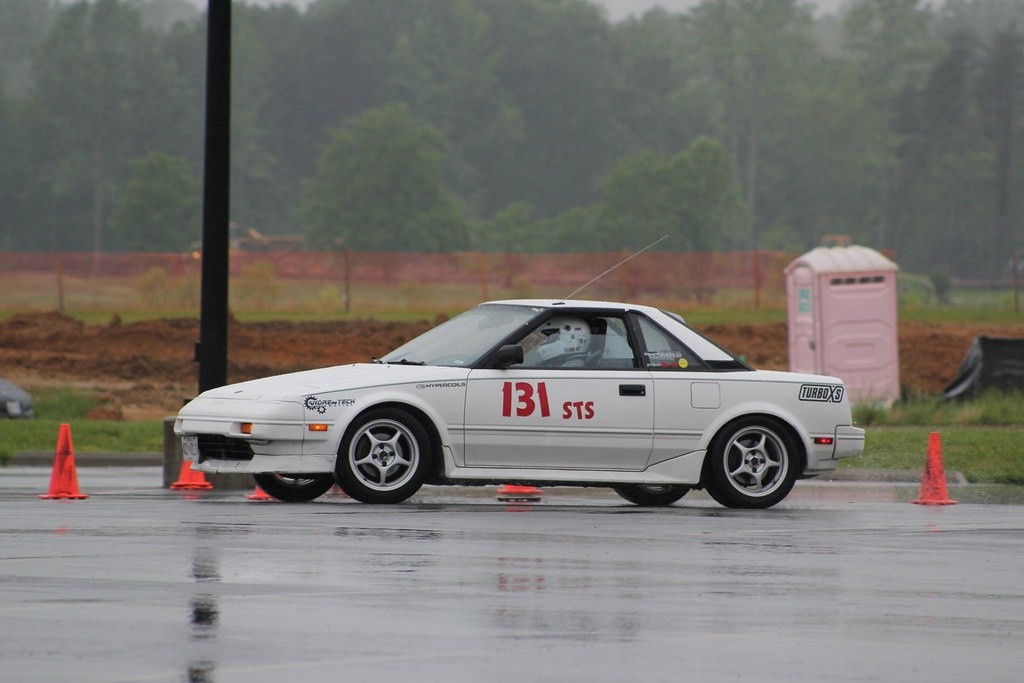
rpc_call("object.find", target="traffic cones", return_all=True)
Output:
[37,422,90,498]
[905,432,959,507]
[245,484,272,501]
[167,456,213,490]
[494,484,543,504]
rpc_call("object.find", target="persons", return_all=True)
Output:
[535,316,590,368]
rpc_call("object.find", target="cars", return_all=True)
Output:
[173,300,865,504]
[0,376,36,422]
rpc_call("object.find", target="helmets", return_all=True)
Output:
[537,317,592,362]
